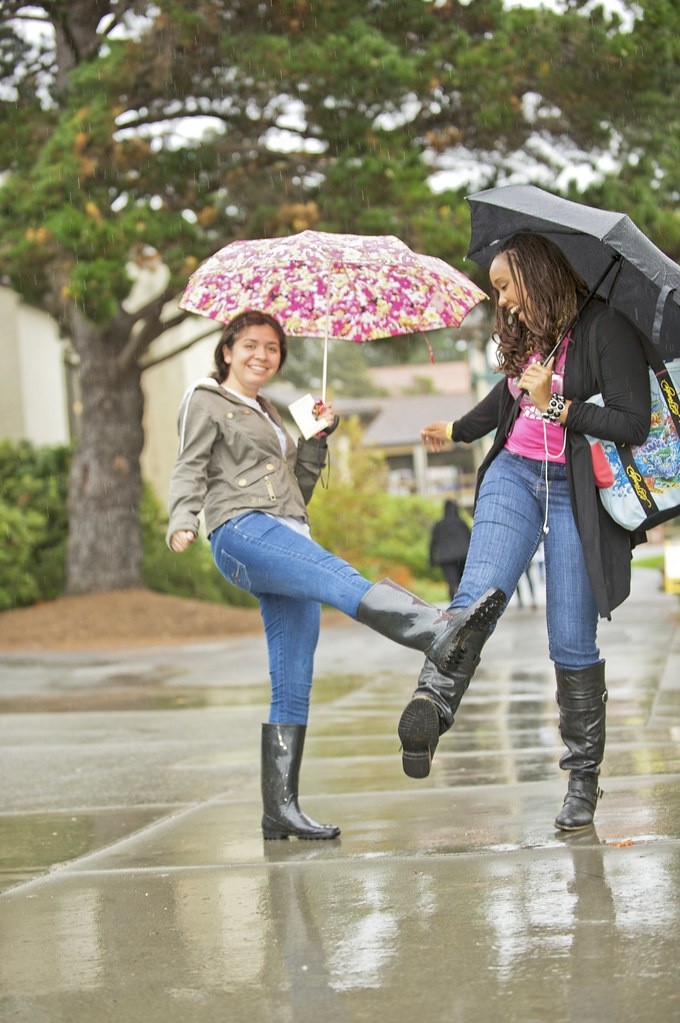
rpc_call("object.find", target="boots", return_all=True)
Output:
[260,722,342,843]
[551,657,609,833]
[396,607,483,782]
[357,575,508,680]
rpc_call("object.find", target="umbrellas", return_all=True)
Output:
[177,229,490,435]
[462,182,680,439]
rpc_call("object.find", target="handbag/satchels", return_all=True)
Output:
[569,362,680,534]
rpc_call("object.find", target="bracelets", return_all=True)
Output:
[543,393,565,424]
[445,421,455,443]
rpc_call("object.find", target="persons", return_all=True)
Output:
[163,311,507,839]
[398,233,654,833]
[428,500,475,601]
